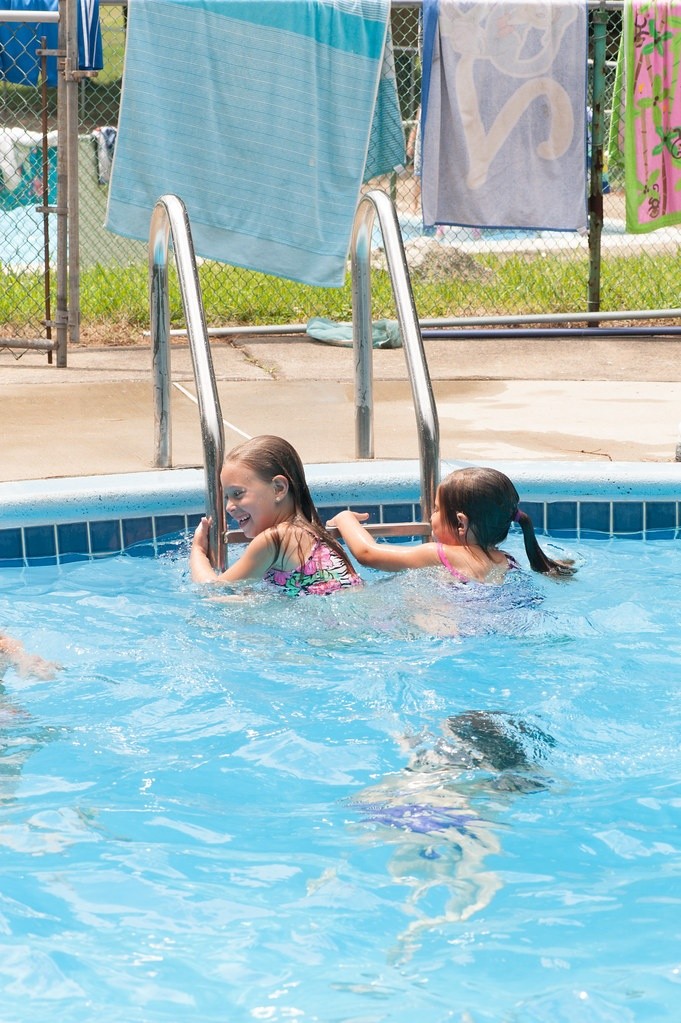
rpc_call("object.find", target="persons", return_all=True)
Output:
[288,711,555,966]
[188,434,364,596]
[325,465,525,585]
[2,630,57,809]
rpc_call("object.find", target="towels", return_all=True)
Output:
[607,0,681,234]
[102,0,410,290]
[0,0,104,90]
[417,0,596,241]
[0,120,115,217]
